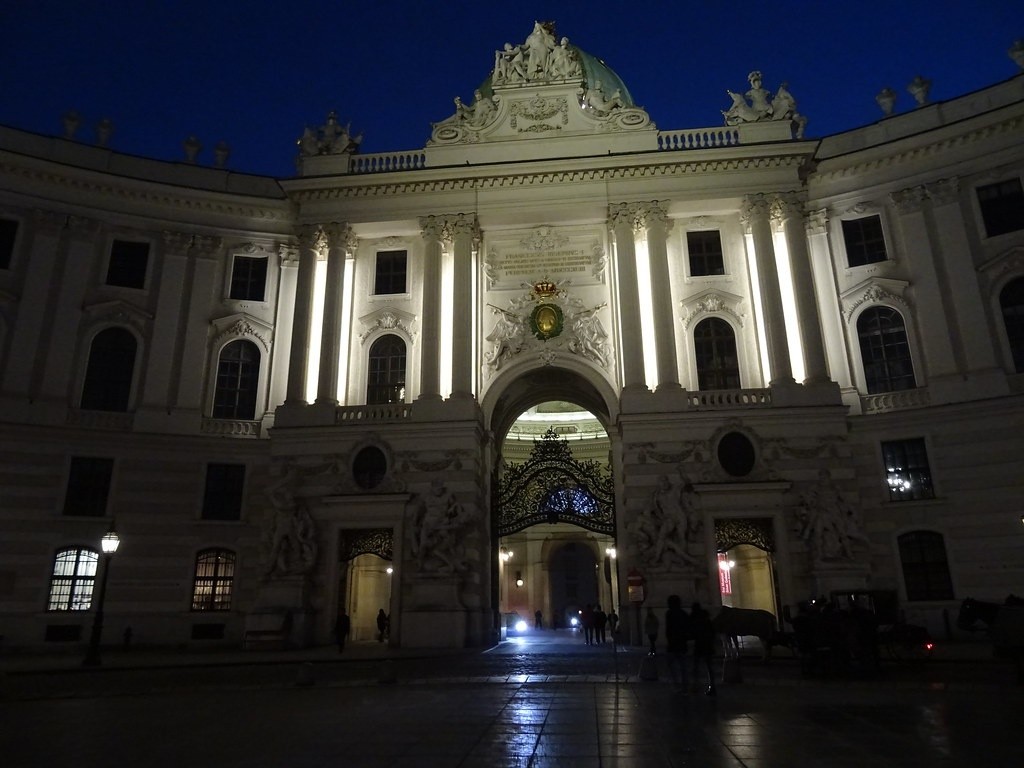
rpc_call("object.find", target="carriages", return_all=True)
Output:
[713,589,933,678]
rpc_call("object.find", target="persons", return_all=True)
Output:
[665,594,740,696]
[797,468,857,563]
[645,609,659,656]
[265,489,304,567]
[377,609,390,642]
[722,71,808,139]
[644,467,689,566]
[411,477,459,571]
[580,604,608,646]
[313,112,346,155]
[487,308,525,370]
[331,607,350,654]
[568,308,607,367]
[453,20,625,111]
[535,610,543,631]
[607,609,618,636]
[553,613,558,631]
[281,611,294,652]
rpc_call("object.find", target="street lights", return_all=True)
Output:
[81,530,121,665]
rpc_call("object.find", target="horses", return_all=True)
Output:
[956,596,1024,676]
[711,606,777,664]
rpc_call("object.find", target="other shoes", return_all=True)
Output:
[596,641,600,645]
[647,650,657,656]
[603,641,606,644]
[705,687,718,696]
[584,641,593,646]
[801,671,819,680]
